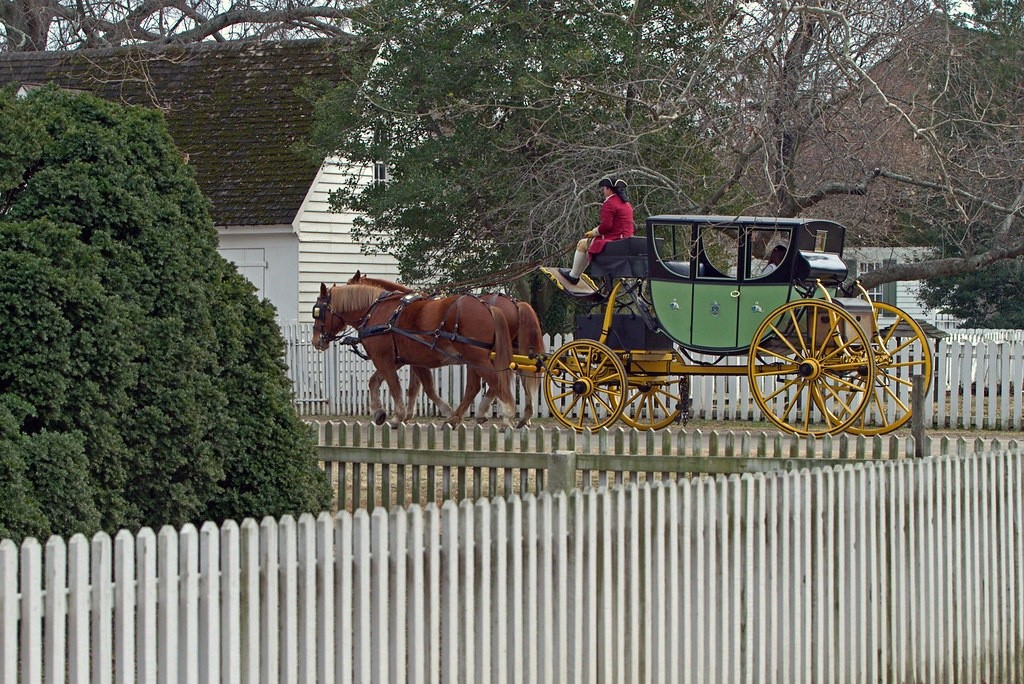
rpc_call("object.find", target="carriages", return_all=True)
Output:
[311,214,934,440]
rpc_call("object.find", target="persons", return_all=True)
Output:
[761,245,787,275]
[558,178,634,285]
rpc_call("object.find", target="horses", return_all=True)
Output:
[312,270,546,428]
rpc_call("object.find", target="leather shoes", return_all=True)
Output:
[559,268,580,285]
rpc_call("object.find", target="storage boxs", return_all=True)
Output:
[806,297,873,345]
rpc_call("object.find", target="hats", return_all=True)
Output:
[599,175,628,188]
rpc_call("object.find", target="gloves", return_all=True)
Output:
[584,230,593,239]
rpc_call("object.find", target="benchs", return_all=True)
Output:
[663,261,706,276]
[591,236,665,277]
